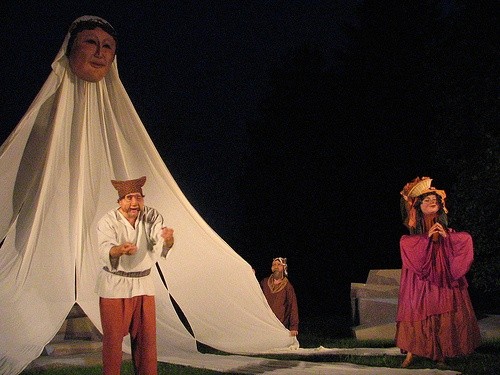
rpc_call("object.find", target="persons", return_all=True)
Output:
[66,17,118,82]
[395,175,482,369]
[252,256,298,336]
[96,175,174,375]
